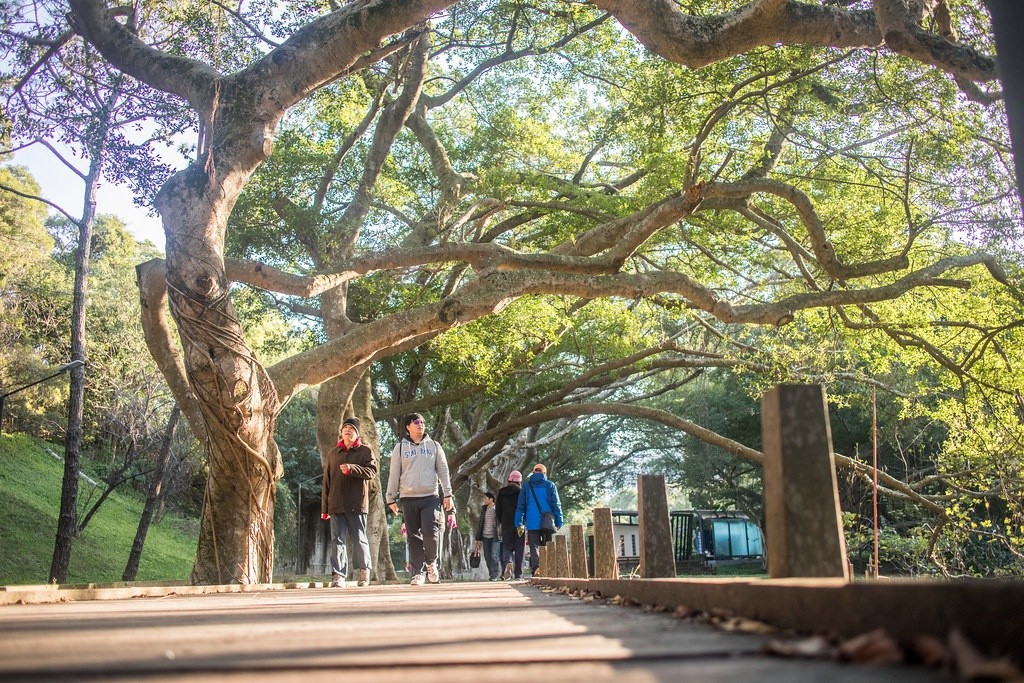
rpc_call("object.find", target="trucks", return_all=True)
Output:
[611,508,767,578]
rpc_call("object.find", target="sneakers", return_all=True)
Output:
[331,573,346,587]
[411,572,426,585]
[426,562,439,583]
[357,569,371,587]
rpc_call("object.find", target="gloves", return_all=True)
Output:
[448,515,457,529]
[402,522,406,536]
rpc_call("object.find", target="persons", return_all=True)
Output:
[321,417,377,587]
[474,463,563,581]
[386,413,457,584]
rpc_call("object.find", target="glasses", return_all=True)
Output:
[413,420,426,425]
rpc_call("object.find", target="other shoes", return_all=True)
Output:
[515,577,525,581]
[532,568,542,577]
[503,563,513,580]
[489,577,499,582]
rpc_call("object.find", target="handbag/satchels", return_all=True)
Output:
[469,551,481,568]
[543,512,559,534]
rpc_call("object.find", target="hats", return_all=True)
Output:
[532,464,547,474]
[507,470,522,483]
[340,417,361,437]
